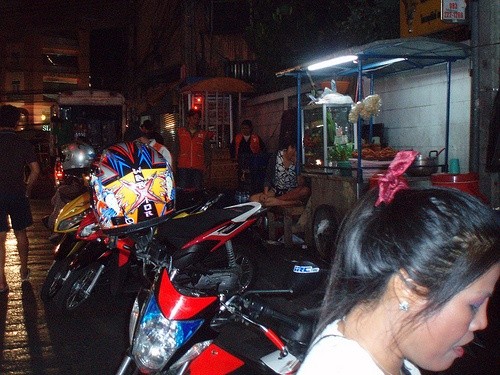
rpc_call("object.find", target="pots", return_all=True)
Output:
[406,150,439,177]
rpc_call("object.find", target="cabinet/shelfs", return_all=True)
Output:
[302,103,357,172]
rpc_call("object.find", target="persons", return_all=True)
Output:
[41,177,84,242]
[0,104,41,293]
[295,175,500,375]
[230,120,266,192]
[124,118,164,146]
[172,109,212,190]
[249,141,312,207]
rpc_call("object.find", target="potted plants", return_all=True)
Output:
[328,144,355,174]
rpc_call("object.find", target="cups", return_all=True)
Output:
[450,159,460,173]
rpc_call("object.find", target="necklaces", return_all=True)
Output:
[342,315,402,375]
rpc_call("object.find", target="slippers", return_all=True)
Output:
[0,284,9,292]
[20,269,29,281]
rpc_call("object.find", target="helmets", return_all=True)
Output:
[62,142,95,170]
[90,140,177,236]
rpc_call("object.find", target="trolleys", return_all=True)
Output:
[278,40,471,269]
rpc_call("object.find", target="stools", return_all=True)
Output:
[267,207,309,249]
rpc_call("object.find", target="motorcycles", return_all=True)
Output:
[40,141,500,375]
